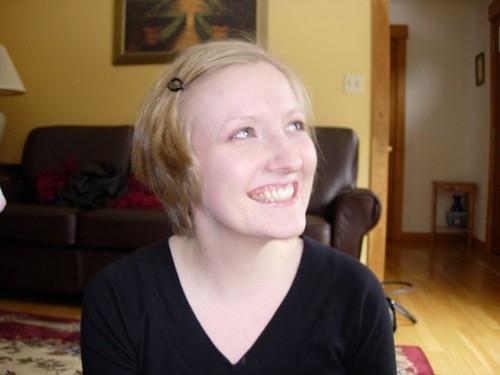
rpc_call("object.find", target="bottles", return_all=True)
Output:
[450,195,464,211]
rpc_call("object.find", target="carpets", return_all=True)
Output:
[0,307,431,375]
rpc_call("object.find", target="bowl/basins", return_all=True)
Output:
[446,211,467,226]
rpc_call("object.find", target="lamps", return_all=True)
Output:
[0,42,25,136]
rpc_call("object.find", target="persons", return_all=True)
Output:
[79,26,397,375]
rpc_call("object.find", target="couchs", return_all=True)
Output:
[0,124,381,306]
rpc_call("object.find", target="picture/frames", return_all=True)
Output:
[112,0,268,66]
[475,51,485,86]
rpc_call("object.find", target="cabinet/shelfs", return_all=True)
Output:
[430,180,477,258]
